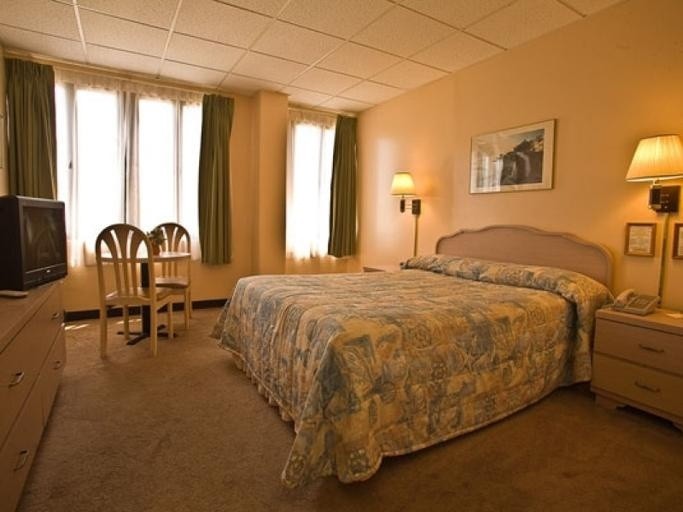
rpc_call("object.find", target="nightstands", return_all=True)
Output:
[362,265,400,272]
[590,305,683,431]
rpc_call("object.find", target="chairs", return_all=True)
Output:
[95,224,176,357]
[150,222,193,330]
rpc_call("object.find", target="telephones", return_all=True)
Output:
[613,289,660,315]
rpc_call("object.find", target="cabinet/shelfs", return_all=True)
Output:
[0,277,67,512]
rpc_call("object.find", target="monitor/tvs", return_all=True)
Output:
[0,194,68,291]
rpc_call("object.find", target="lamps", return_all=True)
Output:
[624,134,683,212]
[390,171,421,214]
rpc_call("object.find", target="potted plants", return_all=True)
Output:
[147,228,163,255]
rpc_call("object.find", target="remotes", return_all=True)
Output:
[0,290,28,297]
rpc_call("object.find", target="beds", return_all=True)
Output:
[208,223,613,492]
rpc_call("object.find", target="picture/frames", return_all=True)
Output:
[468,119,556,195]
[624,222,657,257]
[672,223,683,259]
